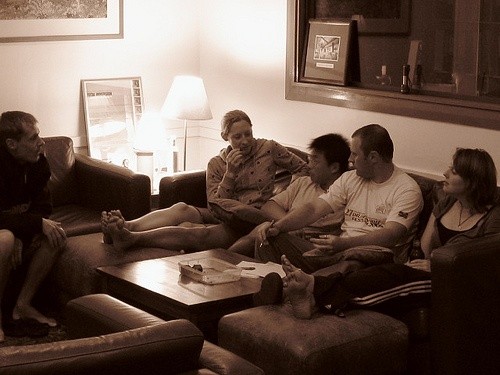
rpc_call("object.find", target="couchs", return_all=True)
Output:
[0,294,264,375]
[0,135,152,313]
[158,147,499,368]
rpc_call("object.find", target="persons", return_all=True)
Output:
[107,133,351,258]
[253,124,424,306]
[102,110,309,250]
[0,111,66,342]
[281,149,500,319]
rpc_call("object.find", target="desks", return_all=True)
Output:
[94,247,304,341]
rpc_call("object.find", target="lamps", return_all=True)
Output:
[163,74,212,172]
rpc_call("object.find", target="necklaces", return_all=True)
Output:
[458,207,477,228]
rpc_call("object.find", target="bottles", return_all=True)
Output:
[410,240,425,261]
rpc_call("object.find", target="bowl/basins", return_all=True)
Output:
[178,257,242,284]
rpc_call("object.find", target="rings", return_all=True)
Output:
[326,235,327,238]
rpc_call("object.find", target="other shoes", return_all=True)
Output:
[254,271,285,308]
[4,319,50,338]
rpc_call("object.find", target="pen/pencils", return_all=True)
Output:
[259,219,275,248]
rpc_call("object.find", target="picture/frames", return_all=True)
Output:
[313,0,412,36]
[81,76,145,167]
[0,0,125,42]
[298,17,359,85]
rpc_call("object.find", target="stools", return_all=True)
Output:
[217,302,409,375]
[54,231,185,308]
